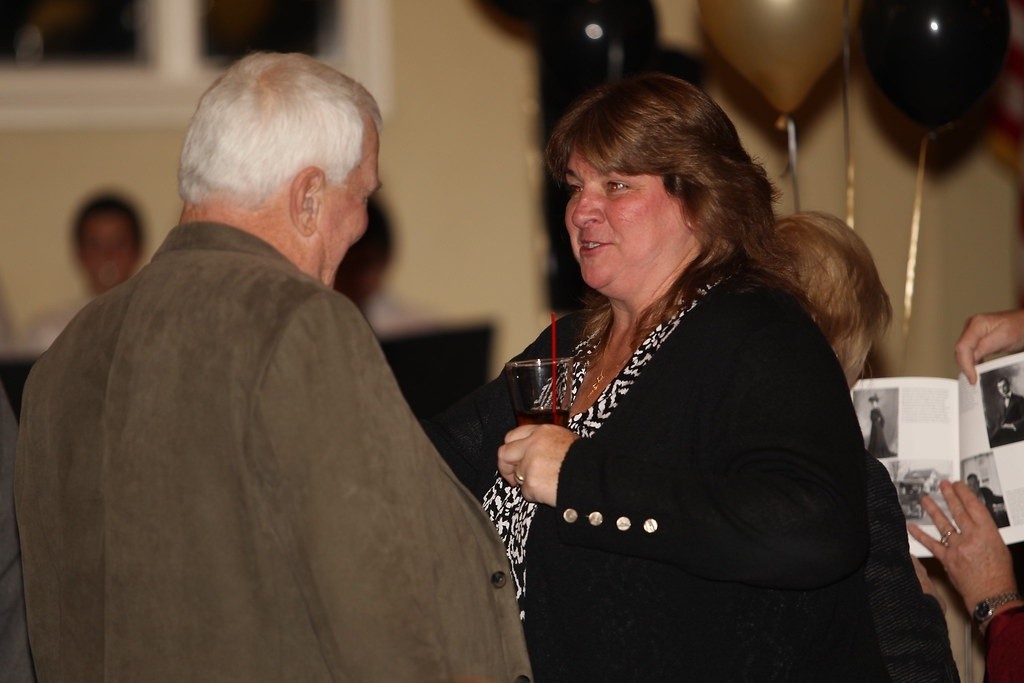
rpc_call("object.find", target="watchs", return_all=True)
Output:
[971,592,1018,631]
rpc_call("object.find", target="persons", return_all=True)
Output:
[965,473,1010,528]
[906,304,1024,683]
[436,73,964,683]
[863,393,896,459]
[986,373,1024,442]
[759,214,894,393]
[29,187,152,352]
[14,52,533,683]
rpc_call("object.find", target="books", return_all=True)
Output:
[850,349,1024,559]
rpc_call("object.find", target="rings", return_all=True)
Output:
[941,528,956,544]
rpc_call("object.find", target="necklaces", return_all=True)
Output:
[584,316,640,397]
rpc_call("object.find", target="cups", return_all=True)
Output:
[505,356,575,428]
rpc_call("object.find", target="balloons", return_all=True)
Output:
[484,0,1013,160]
